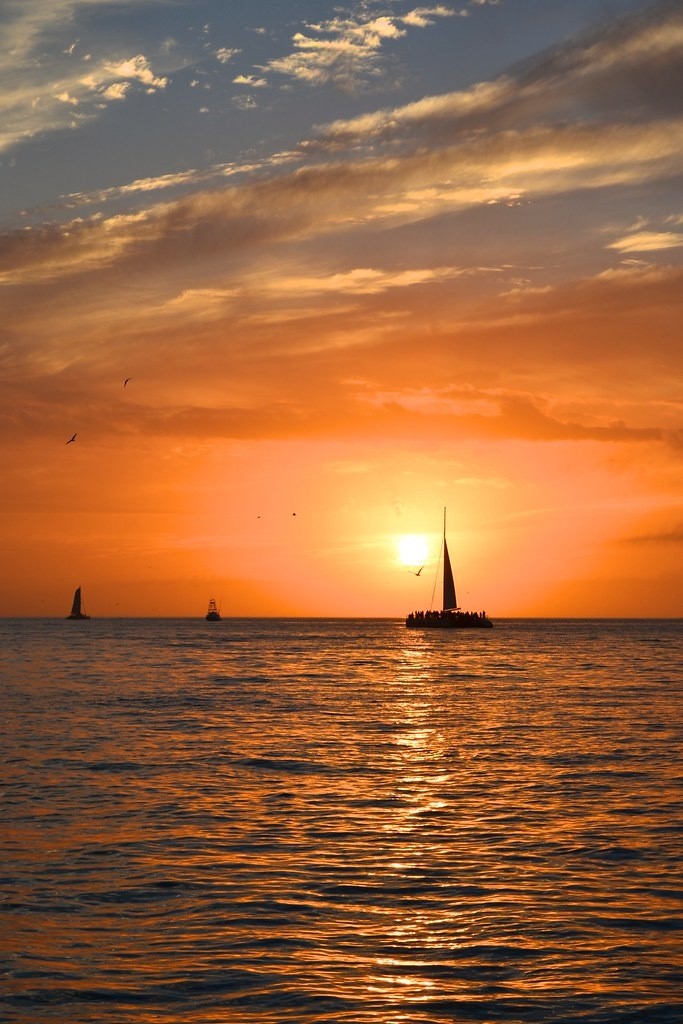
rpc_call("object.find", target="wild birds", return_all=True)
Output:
[66,433,78,445]
[291,512,296,516]
[407,565,424,577]
[257,516,262,520]
[122,377,132,387]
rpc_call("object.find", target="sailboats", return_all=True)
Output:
[406,506,493,627]
[206,598,222,621]
[65,586,90,620]
